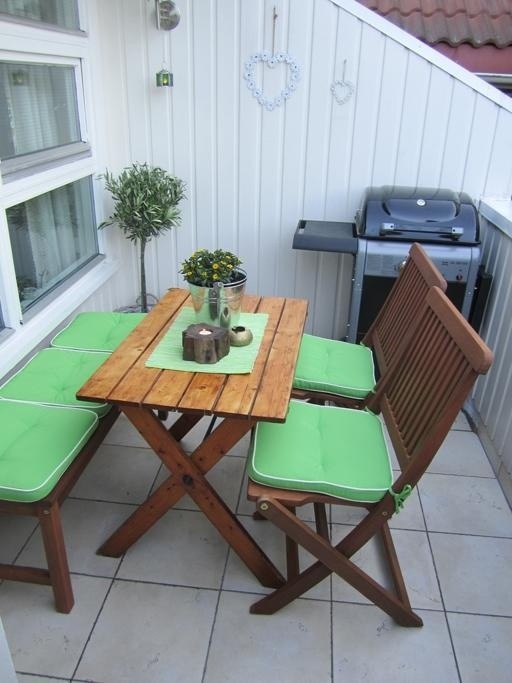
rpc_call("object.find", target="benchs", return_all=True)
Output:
[0,310,151,613]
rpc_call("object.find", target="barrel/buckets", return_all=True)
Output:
[190,267,248,328]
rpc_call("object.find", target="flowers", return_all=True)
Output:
[178,245,244,287]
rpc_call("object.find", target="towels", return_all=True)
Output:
[144,305,269,375]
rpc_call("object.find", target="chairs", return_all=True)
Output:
[291,241,448,410]
[247,284,495,626]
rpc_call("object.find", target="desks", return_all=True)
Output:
[75,288,309,588]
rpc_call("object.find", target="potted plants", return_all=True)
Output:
[94,160,190,313]
